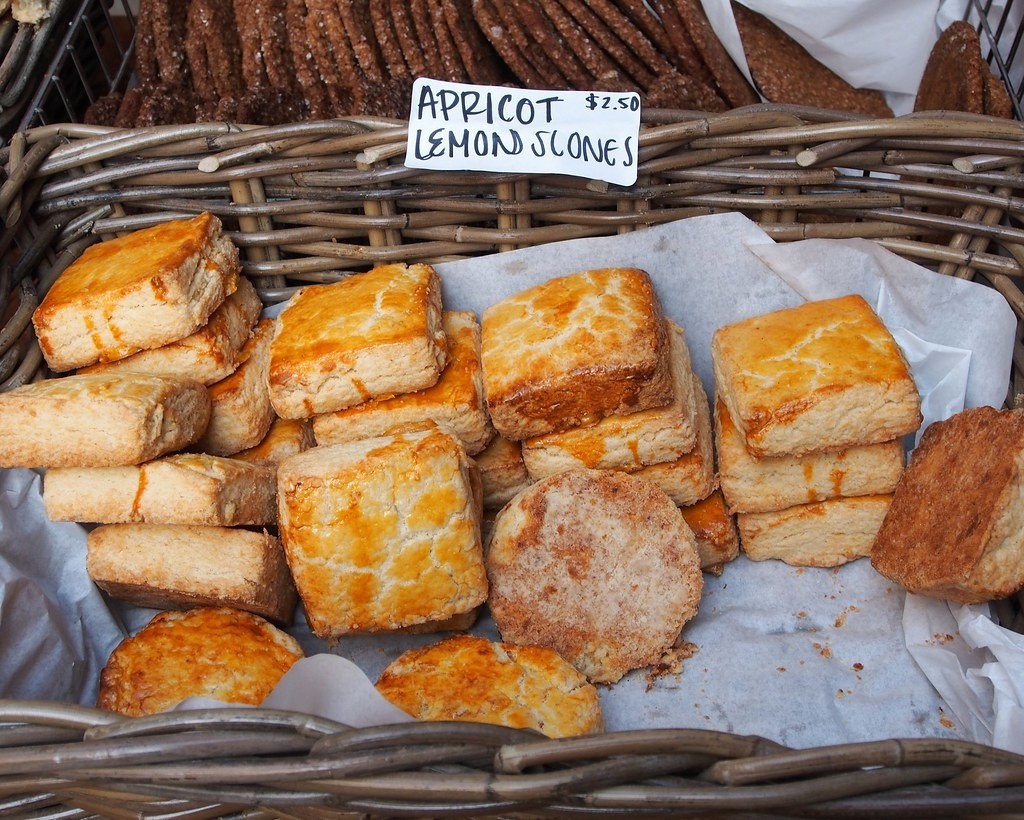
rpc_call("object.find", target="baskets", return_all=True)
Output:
[1,100,1024,819]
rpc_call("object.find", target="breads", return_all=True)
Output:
[1,215,1024,743]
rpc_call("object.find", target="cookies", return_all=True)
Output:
[85,0,1013,154]
